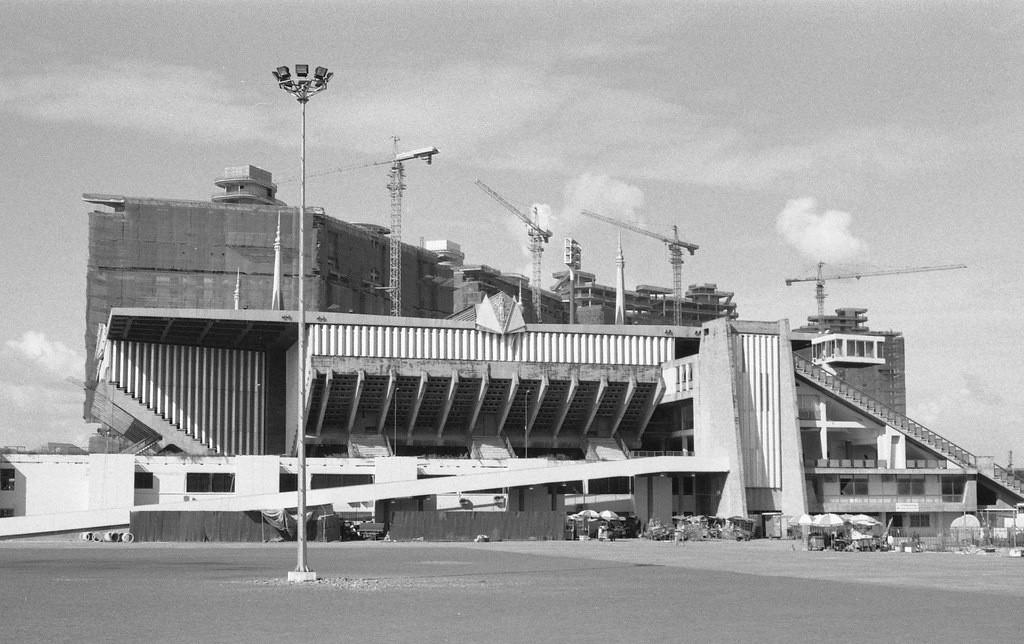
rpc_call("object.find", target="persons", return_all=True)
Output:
[606,519,615,542]
[383,532,391,542]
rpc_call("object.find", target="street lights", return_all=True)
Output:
[270,62,334,583]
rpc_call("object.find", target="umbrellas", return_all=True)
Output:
[787,509,883,553]
[566,510,626,546]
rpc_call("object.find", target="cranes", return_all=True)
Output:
[267,135,439,317]
[473,175,553,321]
[580,208,701,328]
[783,262,970,332]
